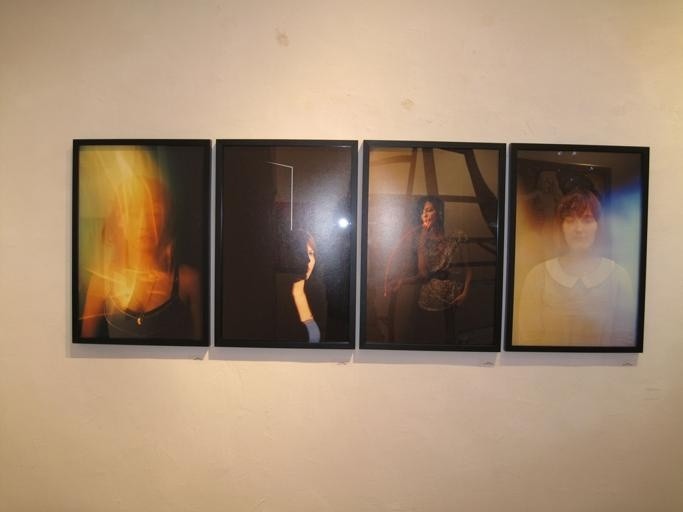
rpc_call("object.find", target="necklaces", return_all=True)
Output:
[132,276,158,325]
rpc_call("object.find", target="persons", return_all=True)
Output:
[81,174,204,341]
[384,194,471,344]
[518,187,637,347]
[273,228,328,343]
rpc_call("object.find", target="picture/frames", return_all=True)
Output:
[502,142,651,354]
[70,138,212,348]
[518,157,611,255]
[359,139,506,352]
[214,138,359,350]
